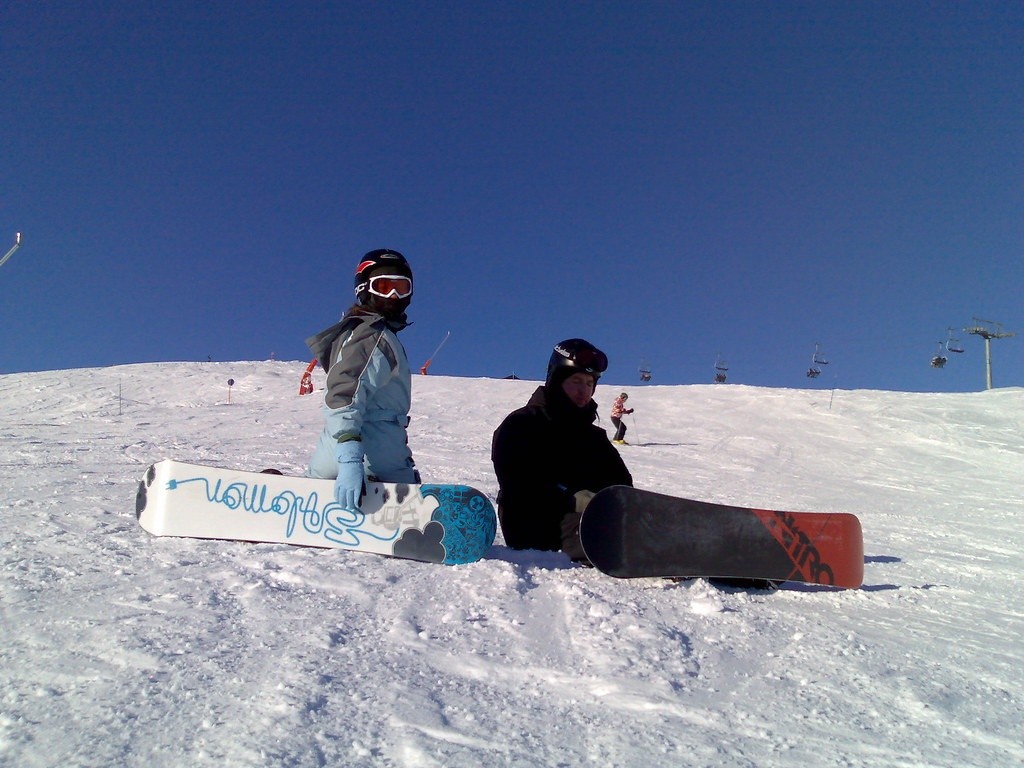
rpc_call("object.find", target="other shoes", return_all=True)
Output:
[612,440,626,444]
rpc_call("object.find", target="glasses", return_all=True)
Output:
[555,345,608,374]
[354,275,412,299]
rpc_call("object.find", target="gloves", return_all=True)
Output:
[627,408,634,414]
[619,409,625,413]
[334,441,367,510]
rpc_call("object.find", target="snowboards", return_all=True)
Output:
[576,483,867,592]
[131,460,498,567]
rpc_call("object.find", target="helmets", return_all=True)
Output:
[546,339,608,390]
[354,249,413,318]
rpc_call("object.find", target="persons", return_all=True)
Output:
[304,249,419,511]
[491,337,634,561]
[609,392,634,443]
